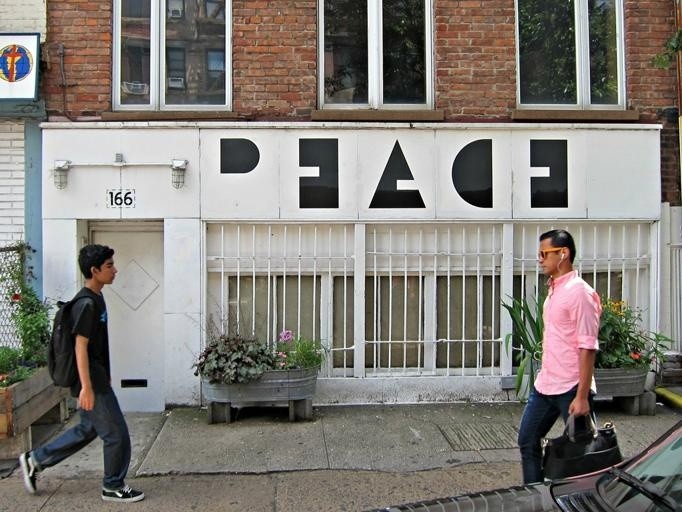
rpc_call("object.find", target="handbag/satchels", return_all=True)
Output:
[543,414,622,479]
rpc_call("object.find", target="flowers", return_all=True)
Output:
[499,287,647,398]
[0,287,57,385]
[191,329,322,385]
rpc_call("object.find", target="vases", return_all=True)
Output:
[0,367,70,438]
[206,363,317,402]
[533,360,647,397]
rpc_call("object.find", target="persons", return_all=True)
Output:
[518,229,602,483]
[20,244,144,503]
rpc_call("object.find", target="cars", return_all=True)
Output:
[362,413,682,512]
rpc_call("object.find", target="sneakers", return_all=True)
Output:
[19,451,38,493]
[102,485,145,503]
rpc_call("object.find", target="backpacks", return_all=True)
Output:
[49,293,100,387]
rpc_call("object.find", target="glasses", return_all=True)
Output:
[540,247,562,258]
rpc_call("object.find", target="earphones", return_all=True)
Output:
[561,253,564,259]
